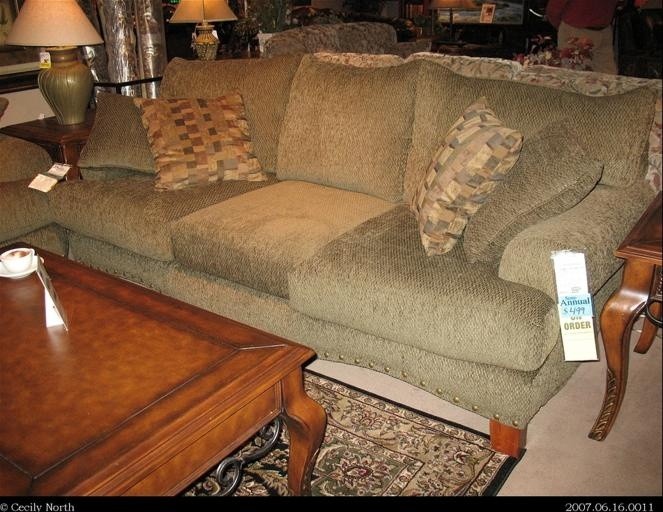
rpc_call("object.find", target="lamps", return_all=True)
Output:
[5,1,105,125]
[168,1,238,60]
[429,1,476,44]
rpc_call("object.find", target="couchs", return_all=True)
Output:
[47,48,662,461]
[263,9,432,60]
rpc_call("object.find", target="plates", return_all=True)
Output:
[0,255,45,280]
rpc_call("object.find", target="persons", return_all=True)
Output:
[544,0,621,78]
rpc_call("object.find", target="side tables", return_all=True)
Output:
[2,109,97,181]
[588,195,662,439]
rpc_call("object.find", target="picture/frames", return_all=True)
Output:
[0,0,101,94]
[480,4,494,23]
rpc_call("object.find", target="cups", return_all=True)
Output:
[0,247,36,274]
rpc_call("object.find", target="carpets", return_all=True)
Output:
[168,368,525,496]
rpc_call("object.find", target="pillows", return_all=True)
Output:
[410,96,603,264]
[76,89,268,192]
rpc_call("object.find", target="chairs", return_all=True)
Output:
[544,0,663,78]
